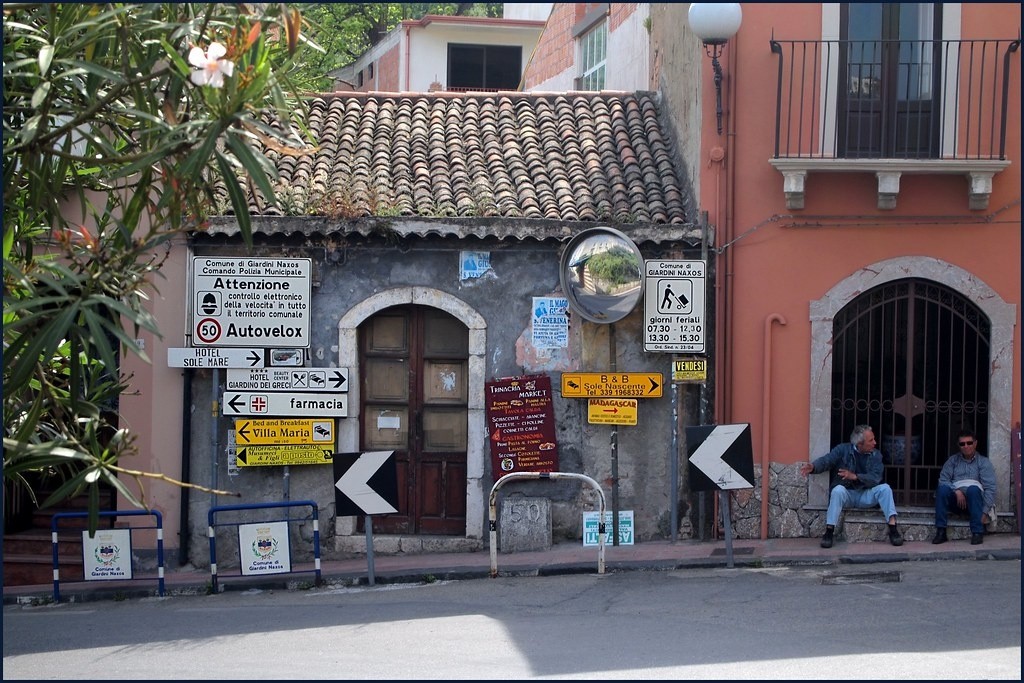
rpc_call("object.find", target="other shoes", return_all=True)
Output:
[931,527,948,544]
[888,522,903,546]
[820,527,834,548]
[971,533,983,545]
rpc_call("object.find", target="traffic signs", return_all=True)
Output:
[168,347,349,468]
[587,398,637,425]
[560,371,664,398]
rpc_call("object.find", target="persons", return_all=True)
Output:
[932,431,997,546]
[800,424,903,548]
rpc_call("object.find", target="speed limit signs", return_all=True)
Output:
[196,318,221,344]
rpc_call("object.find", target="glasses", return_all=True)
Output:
[958,440,975,446]
